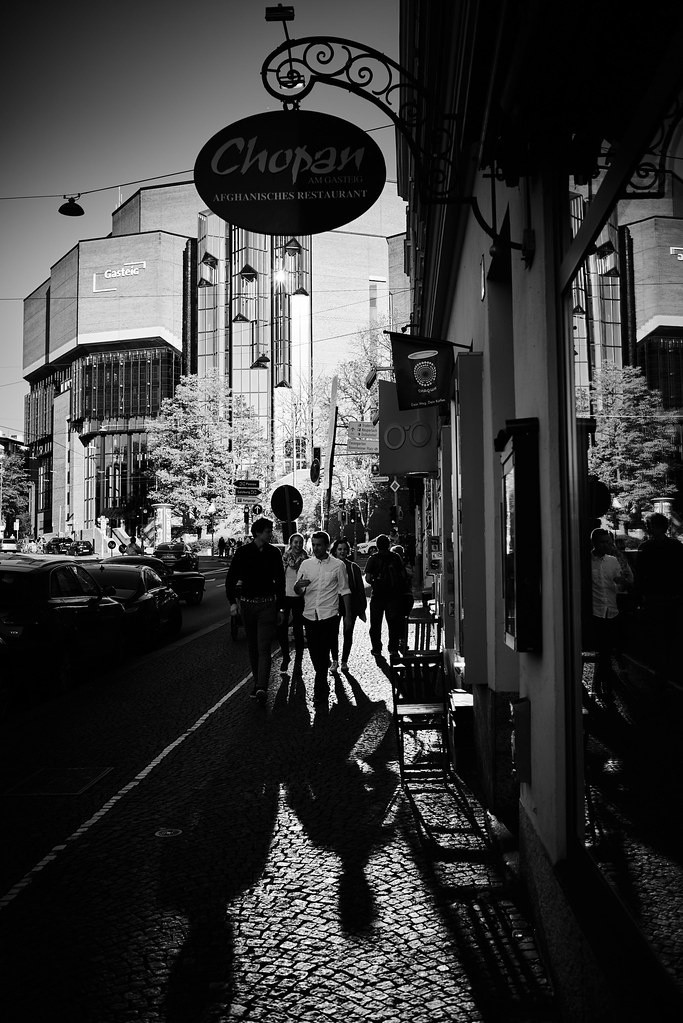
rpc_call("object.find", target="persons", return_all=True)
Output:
[280,533,310,680]
[588,513,683,708]
[363,535,407,657]
[329,540,367,673]
[218,536,255,558]
[225,518,286,710]
[389,545,414,649]
[294,531,352,707]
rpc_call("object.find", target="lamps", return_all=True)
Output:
[258,353,270,361]
[59,192,87,217]
[366,366,379,425]
[279,74,307,89]
[265,2,295,22]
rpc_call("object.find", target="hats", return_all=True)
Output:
[390,545,404,555]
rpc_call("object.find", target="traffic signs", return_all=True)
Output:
[368,476,389,483]
[235,496,262,505]
[346,439,379,454]
[232,479,260,488]
[348,420,379,439]
[234,488,262,496]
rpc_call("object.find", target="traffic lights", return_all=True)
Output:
[349,508,355,524]
[389,505,397,525]
[315,477,322,486]
[244,507,249,524]
[340,510,347,522]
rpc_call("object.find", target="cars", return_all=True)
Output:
[16,537,95,556]
[0,560,128,654]
[152,542,200,572]
[356,537,379,557]
[57,563,183,655]
[99,556,207,608]
[0,538,17,553]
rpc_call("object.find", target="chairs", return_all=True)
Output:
[398,618,445,787]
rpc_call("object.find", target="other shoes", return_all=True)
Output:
[371,649,381,656]
[399,640,407,647]
[256,686,268,700]
[388,646,398,655]
[315,666,328,680]
[294,663,302,674]
[250,685,259,698]
[341,662,349,670]
[280,657,290,671]
[330,660,339,672]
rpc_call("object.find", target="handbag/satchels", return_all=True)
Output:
[359,611,366,622]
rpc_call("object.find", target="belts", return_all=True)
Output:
[240,594,277,603]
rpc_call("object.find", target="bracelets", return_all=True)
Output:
[279,608,285,613]
